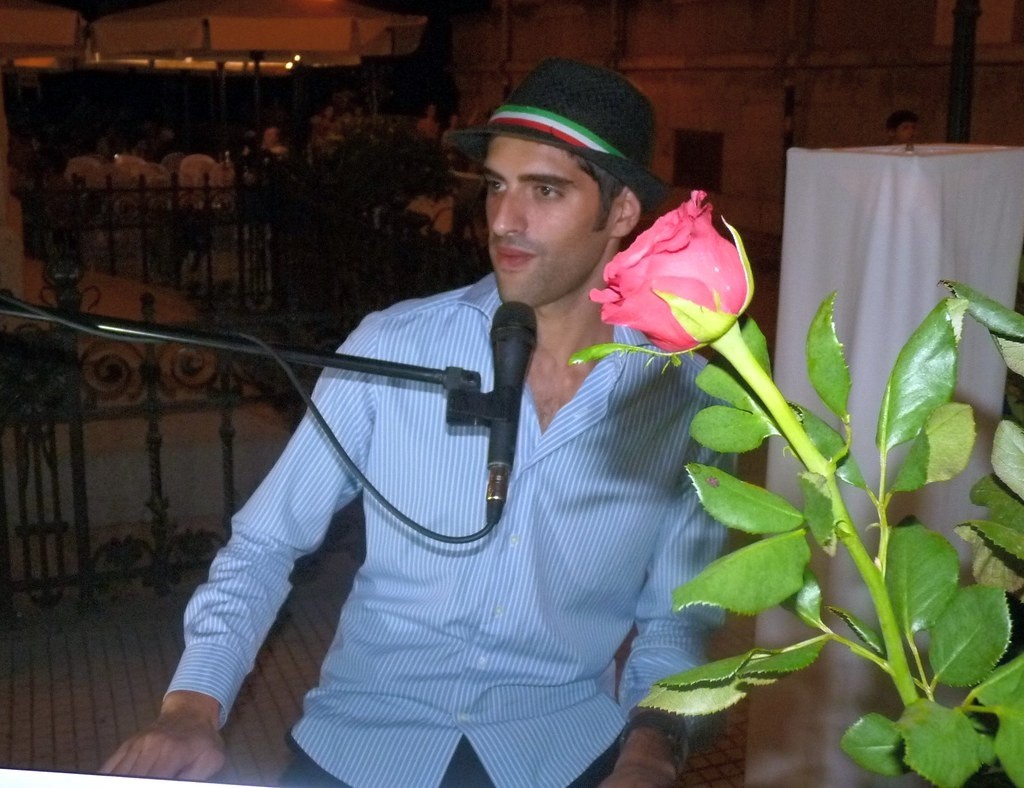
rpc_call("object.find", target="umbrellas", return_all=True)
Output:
[0,0,81,71]
[89,0,428,171]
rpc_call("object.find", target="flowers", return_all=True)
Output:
[573,189,1024,788]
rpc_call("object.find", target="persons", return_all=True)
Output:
[884,109,918,145]
[22,102,490,262]
[98,55,761,788]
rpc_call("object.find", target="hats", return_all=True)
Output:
[449,57,667,210]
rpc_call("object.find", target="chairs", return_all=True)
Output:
[65,151,235,189]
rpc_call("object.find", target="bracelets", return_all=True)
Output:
[619,709,690,777]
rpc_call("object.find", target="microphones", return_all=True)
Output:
[484,301,537,525]
